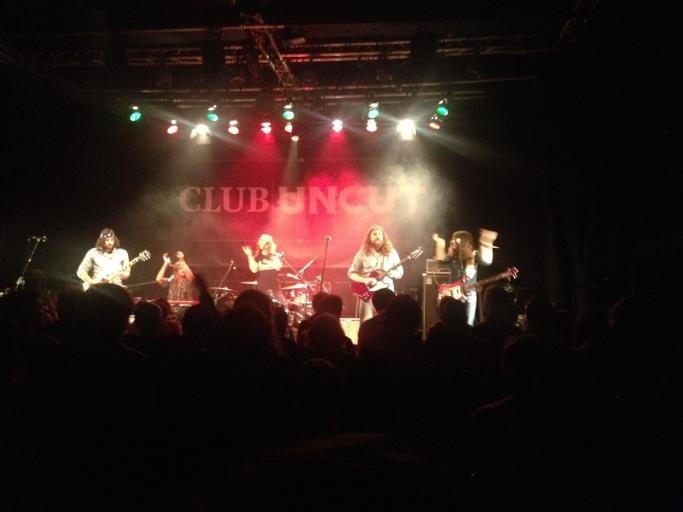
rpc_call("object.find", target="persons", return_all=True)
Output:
[76,228,131,289]
[434,222,498,328]
[243,234,288,311]
[155,250,195,327]
[346,225,404,325]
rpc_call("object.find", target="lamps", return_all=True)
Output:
[126,98,452,147]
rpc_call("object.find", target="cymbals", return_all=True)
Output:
[241,281,258,285]
[211,286,237,292]
[283,283,313,290]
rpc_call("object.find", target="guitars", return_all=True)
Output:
[437,267,518,302]
[350,246,423,304]
[83,250,150,291]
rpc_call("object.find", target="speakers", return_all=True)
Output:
[421,274,465,344]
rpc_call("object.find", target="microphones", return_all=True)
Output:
[31,234,47,244]
[230,260,238,273]
[456,238,463,254]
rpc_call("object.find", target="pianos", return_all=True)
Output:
[147,299,200,307]
